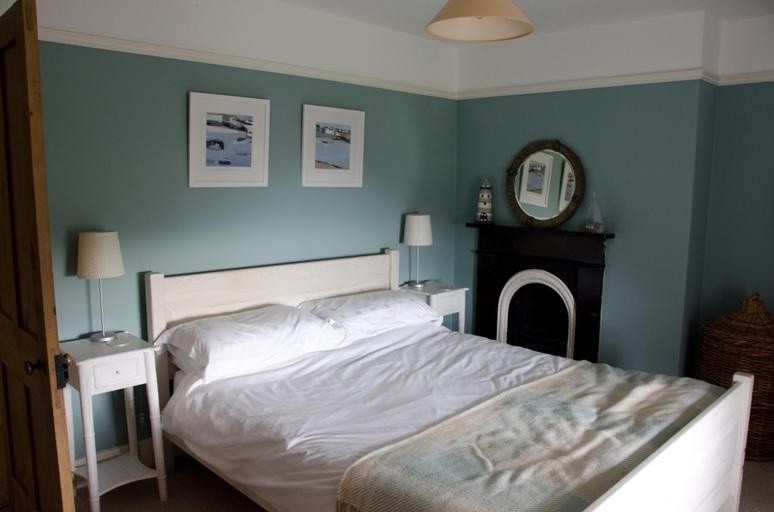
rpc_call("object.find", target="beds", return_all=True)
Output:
[144,249,754,512]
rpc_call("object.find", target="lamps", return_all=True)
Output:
[425,0,535,42]
[403,213,433,288]
[77,230,126,343]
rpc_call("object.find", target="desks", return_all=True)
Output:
[59,332,168,512]
[402,282,470,333]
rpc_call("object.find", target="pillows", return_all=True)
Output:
[154,305,347,383]
[298,290,444,338]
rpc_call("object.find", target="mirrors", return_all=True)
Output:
[506,138,585,229]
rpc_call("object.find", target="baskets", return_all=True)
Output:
[691,293,774,464]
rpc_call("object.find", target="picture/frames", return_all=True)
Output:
[558,160,574,212]
[519,152,553,208]
[302,104,366,187]
[188,92,271,188]
